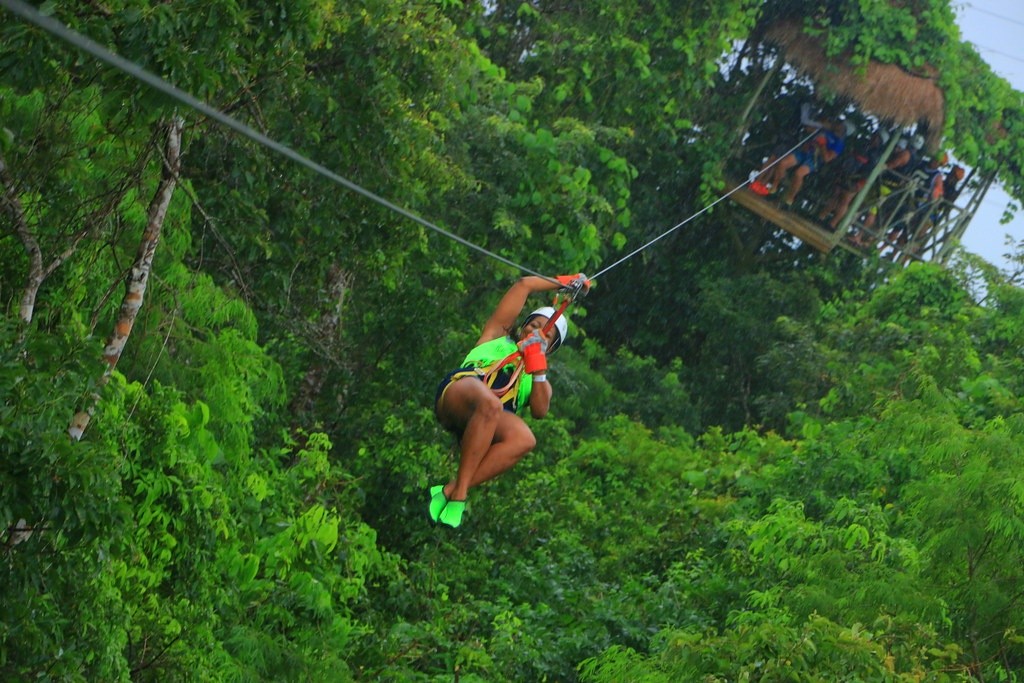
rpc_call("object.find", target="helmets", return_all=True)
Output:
[909,134,925,150]
[934,150,947,165]
[896,138,908,149]
[953,163,964,179]
[844,119,855,136]
[521,307,567,356]
[876,129,890,145]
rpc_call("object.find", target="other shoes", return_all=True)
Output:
[809,216,823,223]
[749,182,769,195]
[781,203,791,213]
[824,223,834,233]
[766,191,778,200]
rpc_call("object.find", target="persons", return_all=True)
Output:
[427,273,590,529]
[751,83,964,265]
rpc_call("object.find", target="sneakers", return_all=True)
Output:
[438,500,466,530]
[427,485,448,529]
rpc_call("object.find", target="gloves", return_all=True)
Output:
[518,328,548,374]
[555,273,590,297]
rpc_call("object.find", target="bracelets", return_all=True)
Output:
[532,374,547,382]
